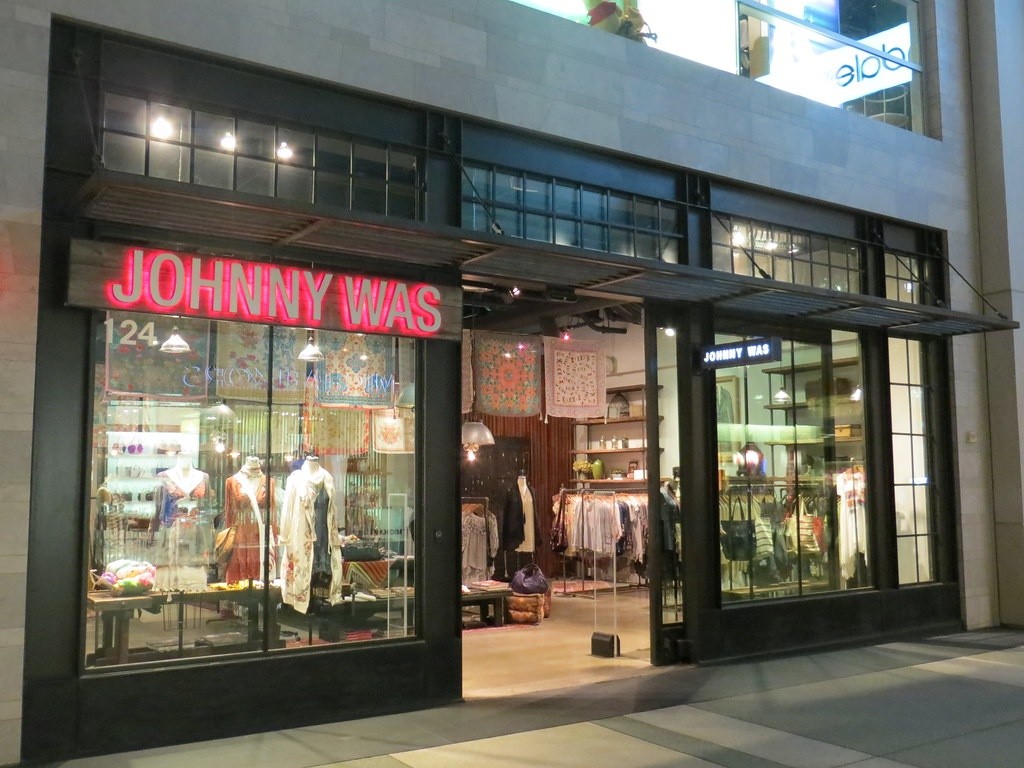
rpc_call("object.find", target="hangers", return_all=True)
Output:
[462,497,491,518]
[779,486,841,516]
[552,488,649,504]
[718,485,777,507]
[846,457,865,475]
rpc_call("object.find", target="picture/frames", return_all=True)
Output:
[713,375,740,462]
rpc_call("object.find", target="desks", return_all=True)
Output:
[344,556,416,634]
[87,581,289,662]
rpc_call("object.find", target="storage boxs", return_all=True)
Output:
[805,377,852,402]
[834,423,862,436]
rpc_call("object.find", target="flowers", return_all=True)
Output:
[572,460,592,473]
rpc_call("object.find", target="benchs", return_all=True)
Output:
[315,581,515,635]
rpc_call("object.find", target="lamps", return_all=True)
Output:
[460,407,495,447]
[848,343,863,402]
[160,317,192,356]
[297,331,326,362]
[773,359,791,402]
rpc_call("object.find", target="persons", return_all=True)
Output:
[147,458,218,585]
[225,457,282,582]
[280,456,344,614]
[502,476,542,552]
[660,478,680,551]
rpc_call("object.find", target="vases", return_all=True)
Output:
[579,471,588,480]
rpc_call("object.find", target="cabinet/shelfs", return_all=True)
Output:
[762,356,867,477]
[569,384,672,484]
